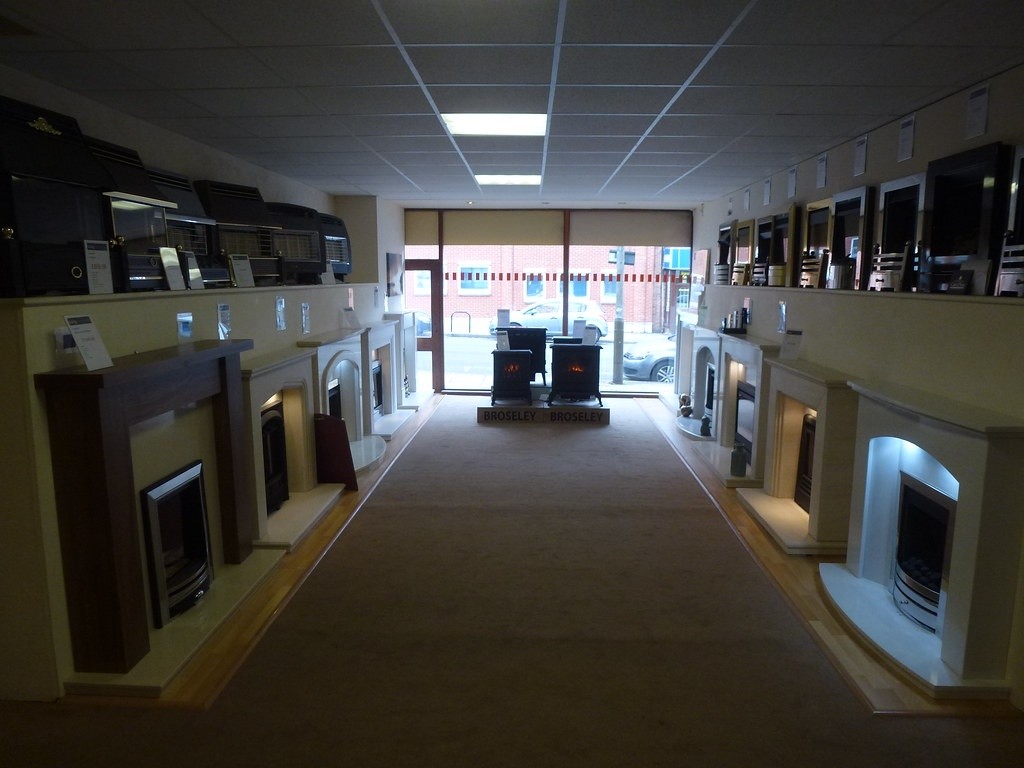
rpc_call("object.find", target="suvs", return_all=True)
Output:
[488,297,609,344]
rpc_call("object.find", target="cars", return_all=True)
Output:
[623,334,675,383]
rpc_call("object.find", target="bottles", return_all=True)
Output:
[729,443,747,477]
